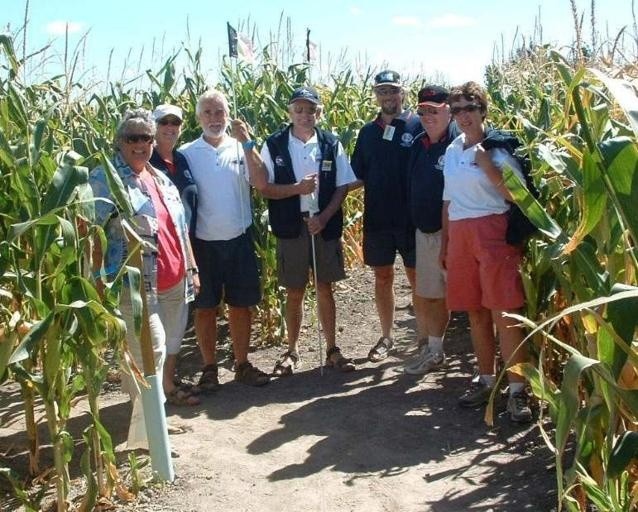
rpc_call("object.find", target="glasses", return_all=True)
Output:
[159,119,181,126]
[126,133,154,144]
[294,104,317,114]
[377,86,399,96]
[417,107,438,115]
[450,104,480,113]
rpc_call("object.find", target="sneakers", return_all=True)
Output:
[404,347,449,375]
[456,377,500,406]
[233,362,270,387]
[506,388,533,423]
[198,364,220,392]
[368,337,394,362]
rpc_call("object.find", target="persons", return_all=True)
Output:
[440,82,532,422]
[404,86,499,384]
[259,88,357,376]
[149,104,202,395]
[349,71,431,362]
[89,108,201,458]
[175,89,270,391]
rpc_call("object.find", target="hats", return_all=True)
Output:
[417,85,449,108]
[373,70,402,88]
[152,104,183,123]
[289,87,320,105]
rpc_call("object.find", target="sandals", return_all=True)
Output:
[167,387,200,406]
[175,382,203,394]
[273,350,301,376]
[324,346,356,372]
